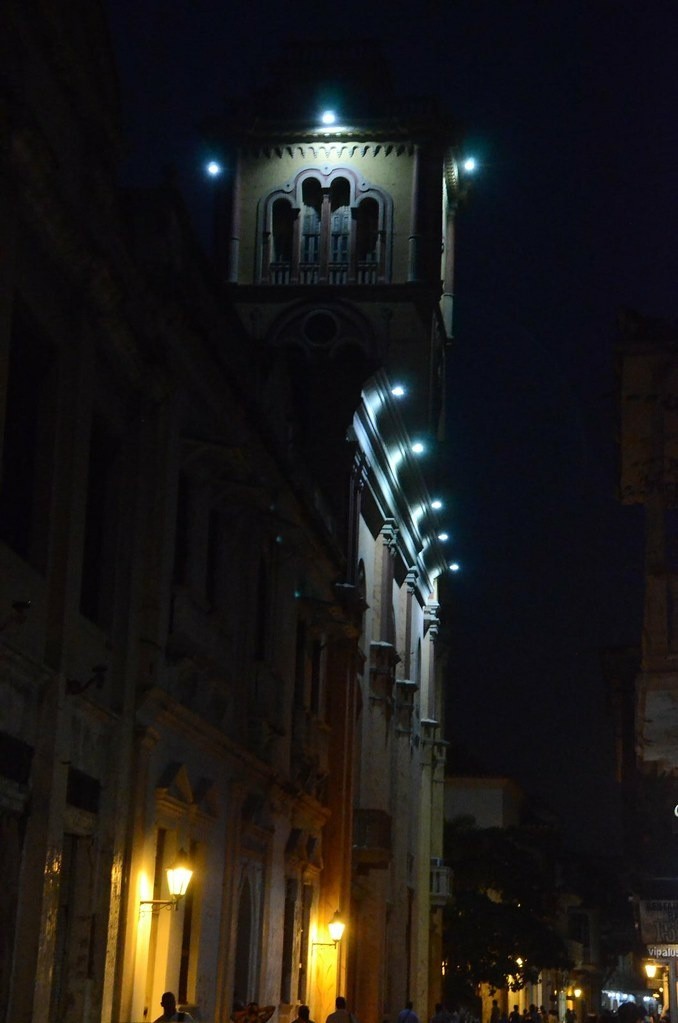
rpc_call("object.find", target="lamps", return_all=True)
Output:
[137,859,194,911]
[311,912,345,948]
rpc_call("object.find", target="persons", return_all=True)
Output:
[325,997,356,1023]
[231,1002,276,1023]
[397,1001,418,1023]
[291,1005,315,1023]
[430,1003,450,1023]
[153,991,194,1023]
[490,999,671,1023]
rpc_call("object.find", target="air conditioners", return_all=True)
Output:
[430,858,445,867]
